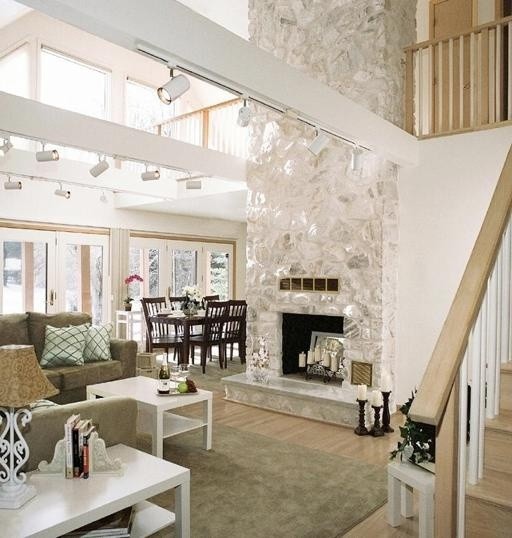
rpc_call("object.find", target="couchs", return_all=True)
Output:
[0,311,138,407]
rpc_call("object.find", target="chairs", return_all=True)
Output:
[140,292,249,372]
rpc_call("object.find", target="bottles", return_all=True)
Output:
[157,352,169,393]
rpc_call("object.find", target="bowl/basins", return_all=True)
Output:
[161,308,171,312]
[197,309,206,314]
[173,310,184,315]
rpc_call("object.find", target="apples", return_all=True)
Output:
[178,383,188,393]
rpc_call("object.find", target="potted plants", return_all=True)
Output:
[124,299,133,311]
[384,379,488,474]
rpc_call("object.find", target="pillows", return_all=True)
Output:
[37,323,90,370]
[68,321,116,362]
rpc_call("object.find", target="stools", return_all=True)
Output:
[386,451,436,538]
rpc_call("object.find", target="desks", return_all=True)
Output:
[116,309,144,345]
[0,441,192,538]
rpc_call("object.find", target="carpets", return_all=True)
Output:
[134,411,390,538]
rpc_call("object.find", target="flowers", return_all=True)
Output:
[181,289,203,301]
[125,273,143,282]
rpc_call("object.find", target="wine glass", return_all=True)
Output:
[172,300,194,316]
[170,363,189,393]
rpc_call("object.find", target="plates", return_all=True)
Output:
[157,312,173,315]
[193,314,211,317]
[166,314,187,318]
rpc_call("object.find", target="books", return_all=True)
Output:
[56,506,135,538]
[63,414,96,480]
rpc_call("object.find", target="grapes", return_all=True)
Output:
[186,379,197,392]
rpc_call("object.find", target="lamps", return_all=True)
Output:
[156,61,331,155]
[0,343,61,510]
[0,132,206,192]
[2,174,109,204]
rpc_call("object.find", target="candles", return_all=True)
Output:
[372,387,382,406]
[381,374,392,393]
[357,382,368,400]
[298,344,339,373]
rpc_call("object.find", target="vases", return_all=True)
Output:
[127,284,142,301]
